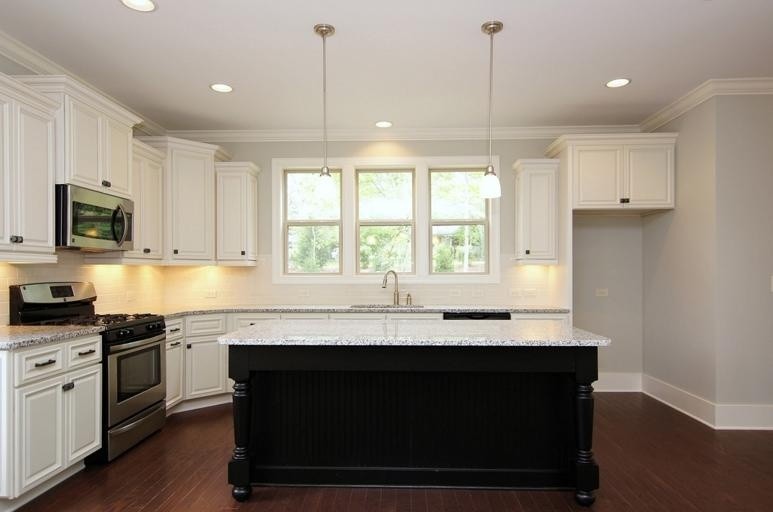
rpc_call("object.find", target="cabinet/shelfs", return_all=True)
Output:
[543,135,676,217]
[166,315,226,410]
[512,159,560,265]
[0,332,102,512]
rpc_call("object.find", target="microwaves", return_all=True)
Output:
[66,184,133,250]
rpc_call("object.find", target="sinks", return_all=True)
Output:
[351,305,424,309]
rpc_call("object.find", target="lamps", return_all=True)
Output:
[478,21,503,200]
[312,22,336,198]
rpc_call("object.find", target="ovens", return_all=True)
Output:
[105,333,166,427]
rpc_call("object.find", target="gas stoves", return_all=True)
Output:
[24,306,164,329]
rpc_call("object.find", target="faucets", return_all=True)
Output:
[382,270,400,305]
